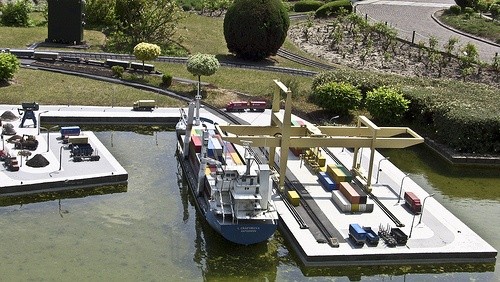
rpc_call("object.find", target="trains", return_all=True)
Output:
[1,47,155,72]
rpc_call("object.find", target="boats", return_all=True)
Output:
[175,94,279,245]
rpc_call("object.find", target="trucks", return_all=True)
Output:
[404,192,422,214]
[60,126,93,156]
[390,227,408,246]
[349,224,379,245]
[133,99,155,111]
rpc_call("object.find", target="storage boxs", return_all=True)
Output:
[287,121,374,215]
[188,128,244,183]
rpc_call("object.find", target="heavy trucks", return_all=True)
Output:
[225,101,266,112]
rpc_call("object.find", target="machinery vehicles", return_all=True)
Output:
[15,134,38,151]
